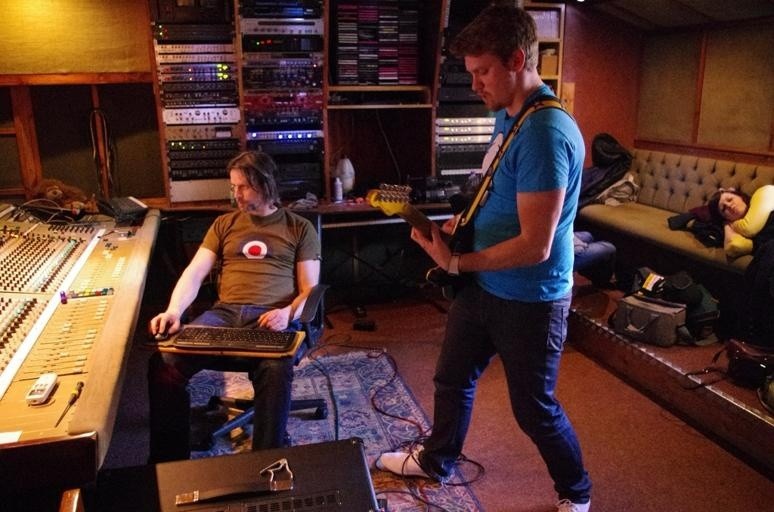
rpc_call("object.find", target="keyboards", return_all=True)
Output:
[173,327,300,352]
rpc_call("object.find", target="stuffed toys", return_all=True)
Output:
[25,178,100,215]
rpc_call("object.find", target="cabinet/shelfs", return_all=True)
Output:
[145,2,567,214]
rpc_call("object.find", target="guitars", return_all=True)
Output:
[367,183,469,299]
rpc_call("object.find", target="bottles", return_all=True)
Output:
[467,170,477,181]
[336,153,356,195]
[334,175,343,202]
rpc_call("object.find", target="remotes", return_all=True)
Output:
[25,372,58,406]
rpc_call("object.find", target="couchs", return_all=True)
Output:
[578,140,772,310]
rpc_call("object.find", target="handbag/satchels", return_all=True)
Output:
[615,291,686,349]
[726,338,772,386]
[661,270,705,310]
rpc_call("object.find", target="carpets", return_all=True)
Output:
[184,348,479,512]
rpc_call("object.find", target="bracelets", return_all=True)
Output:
[447,250,460,278]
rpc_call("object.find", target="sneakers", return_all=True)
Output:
[556,497,591,512]
[379,451,432,477]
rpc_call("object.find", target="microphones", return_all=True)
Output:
[247,199,265,213]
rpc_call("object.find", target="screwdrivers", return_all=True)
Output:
[54,381,84,427]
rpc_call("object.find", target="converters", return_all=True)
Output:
[353,320,375,330]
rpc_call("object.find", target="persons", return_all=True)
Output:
[375,7,594,511]
[146,152,325,467]
[460,172,617,286]
[709,183,773,291]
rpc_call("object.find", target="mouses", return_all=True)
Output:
[153,331,169,341]
